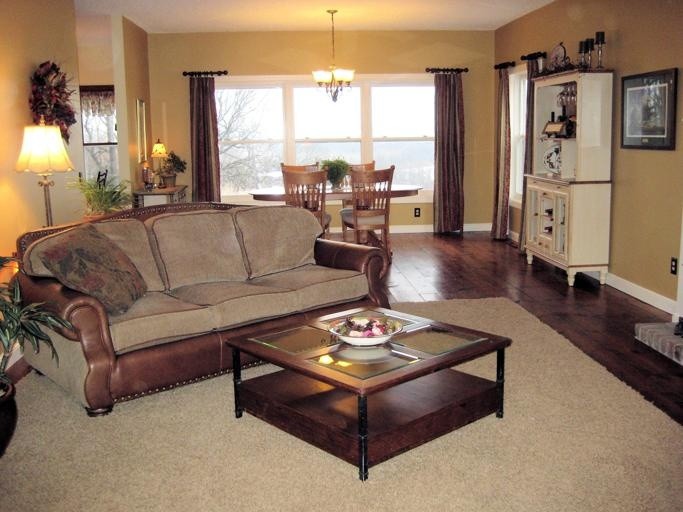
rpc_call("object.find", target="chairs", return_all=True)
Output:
[281,167,331,240]
[338,160,376,240]
[279,160,319,211]
[339,165,394,263]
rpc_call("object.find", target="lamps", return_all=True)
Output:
[312,9,355,103]
[150,137,169,188]
[14,114,77,223]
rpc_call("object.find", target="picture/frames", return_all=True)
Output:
[79,83,117,146]
[620,67,680,152]
[134,97,148,164]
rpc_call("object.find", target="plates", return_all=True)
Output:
[542,143,561,175]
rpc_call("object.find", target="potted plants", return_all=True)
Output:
[159,152,188,187]
[0,273,76,459]
[320,156,349,192]
[67,173,135,219]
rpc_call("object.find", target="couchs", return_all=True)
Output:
[9,199,391,416]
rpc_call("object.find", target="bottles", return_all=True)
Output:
[549,105,575,138]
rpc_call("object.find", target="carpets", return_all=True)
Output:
[0,298,683,510]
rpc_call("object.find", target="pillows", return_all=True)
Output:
[43,220,147,318]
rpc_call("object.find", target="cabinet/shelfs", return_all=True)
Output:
[132,183,188,206]
[522,175,612,289]
[528,71,615,182]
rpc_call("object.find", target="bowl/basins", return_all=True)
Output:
[329,342,398,365]
[330,320,403,345]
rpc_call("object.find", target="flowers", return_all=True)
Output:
[25,60,76,144]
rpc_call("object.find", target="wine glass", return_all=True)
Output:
[554,84,577,106]
